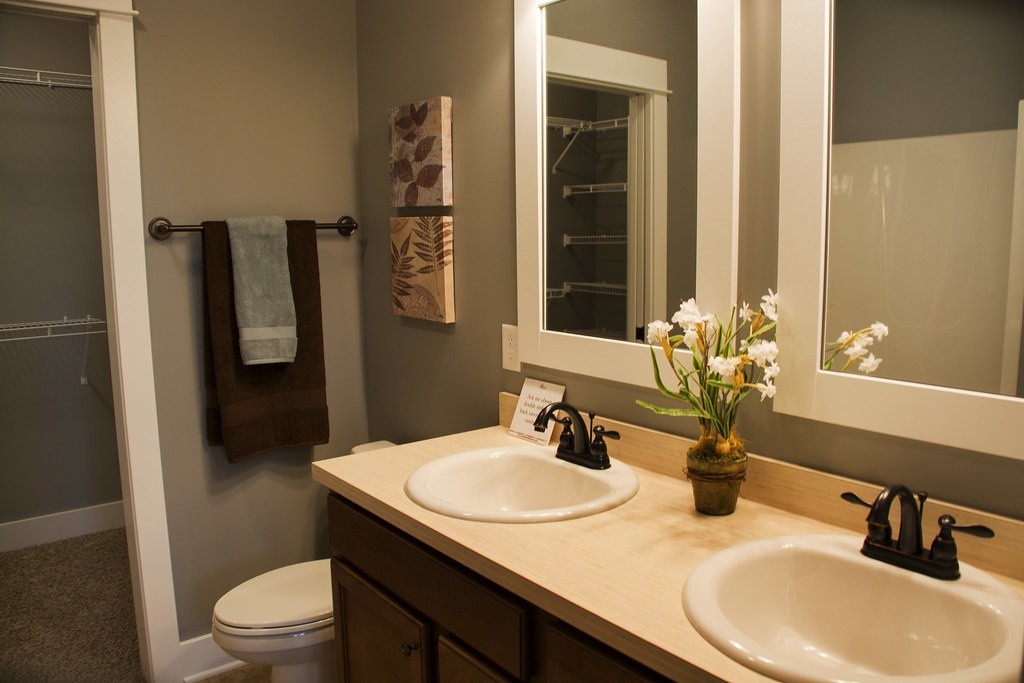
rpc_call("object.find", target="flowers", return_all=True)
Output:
[822,321,889,374]
[634,287,780,459]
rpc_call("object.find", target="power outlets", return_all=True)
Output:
[502,323,521,373]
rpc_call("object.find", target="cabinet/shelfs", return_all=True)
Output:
[325,487,674,682]
[546,116,628,299]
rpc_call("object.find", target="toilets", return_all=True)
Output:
[210,440,398,683]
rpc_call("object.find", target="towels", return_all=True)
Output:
[200,213,331,466]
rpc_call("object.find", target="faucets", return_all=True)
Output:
[533,401,590,457]
[864,482,923,556]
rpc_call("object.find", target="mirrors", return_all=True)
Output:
[514,0,741,409]
[772,0,1024,463]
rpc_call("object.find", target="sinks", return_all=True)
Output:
[682,533,1024,683]
[403,445,639,524]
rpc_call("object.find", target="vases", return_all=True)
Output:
[684,433,747,518]
